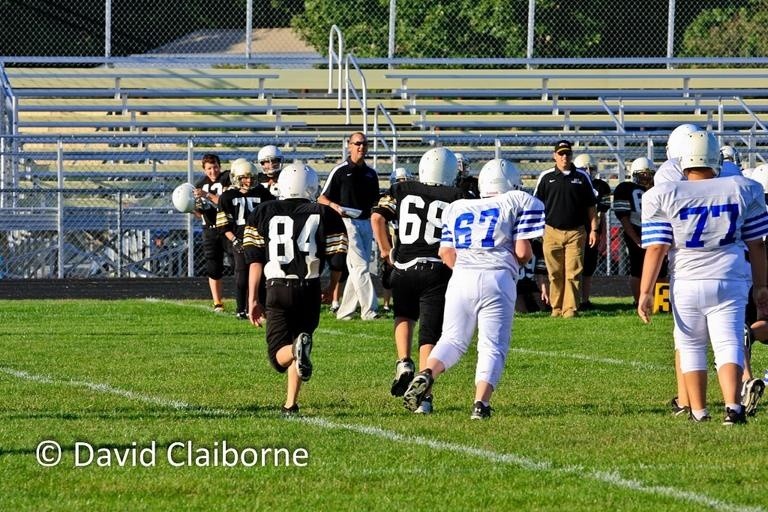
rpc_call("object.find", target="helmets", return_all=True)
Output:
[277,163,320,202]
[172,183,198,214]
[574,154,597,178]
[666,123,737,177]
[478,158,522,198]
[631,157,657,188]
[741,163,768,194]
[257,145,284,178]
[390,147,473,187]
[230,158,259,190]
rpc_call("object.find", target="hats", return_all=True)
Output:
[554,140,571,152]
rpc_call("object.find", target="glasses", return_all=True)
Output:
[350,141,367,146]
[558,150,572,156]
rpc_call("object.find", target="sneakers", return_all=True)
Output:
[470,402,491,420]
[213,303,226,313]
[281,402,299,414]
[391,358,434,415]
[552,300,595,319]
[670,376,765,427]
[236,311,248,320]
[292,332,313,382]
[330,304,390,322]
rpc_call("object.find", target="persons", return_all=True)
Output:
[191,135,768,321]
[242,163,347,414]
[745,318,768,351]
[652,122,768,417]
[370,147,474,414]
[403,158,546,421]
[636,131,768,425]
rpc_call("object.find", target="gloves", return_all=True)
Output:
[231,236,244,254]
